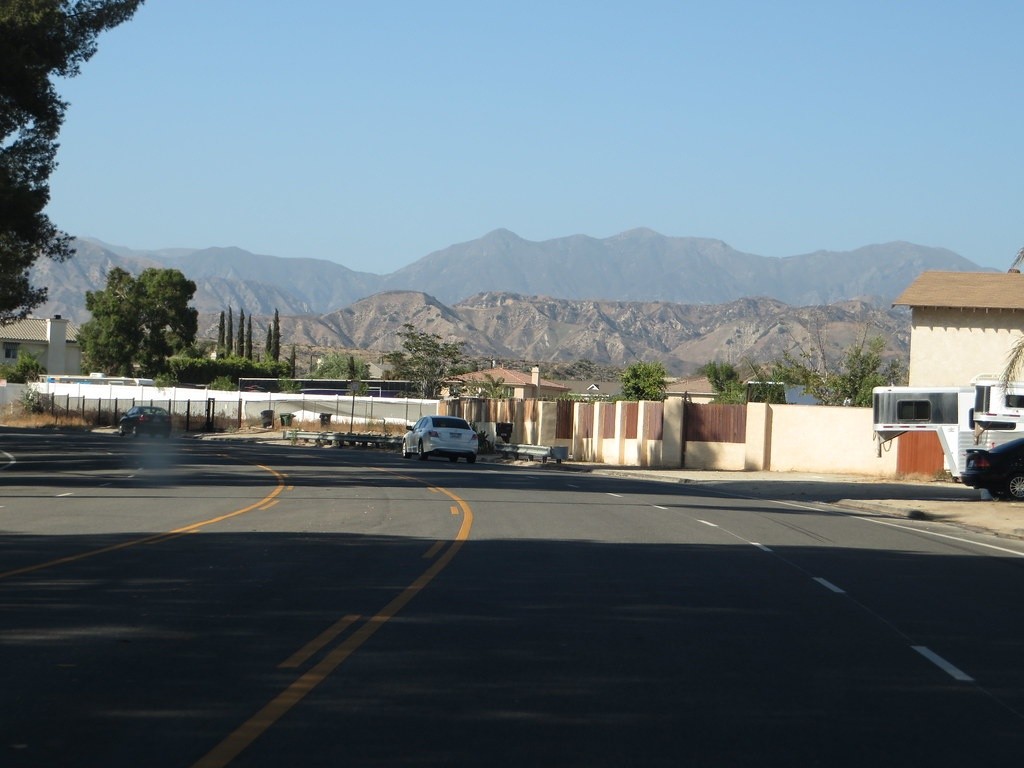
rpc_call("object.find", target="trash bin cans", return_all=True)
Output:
[280,413,295,427]
[320,412,332,427]
[261,410,275,428]
[496,423,513,443]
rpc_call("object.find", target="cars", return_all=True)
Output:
[960,437,1024,502]
[401,415,478,464]
[118,406,172,437]
